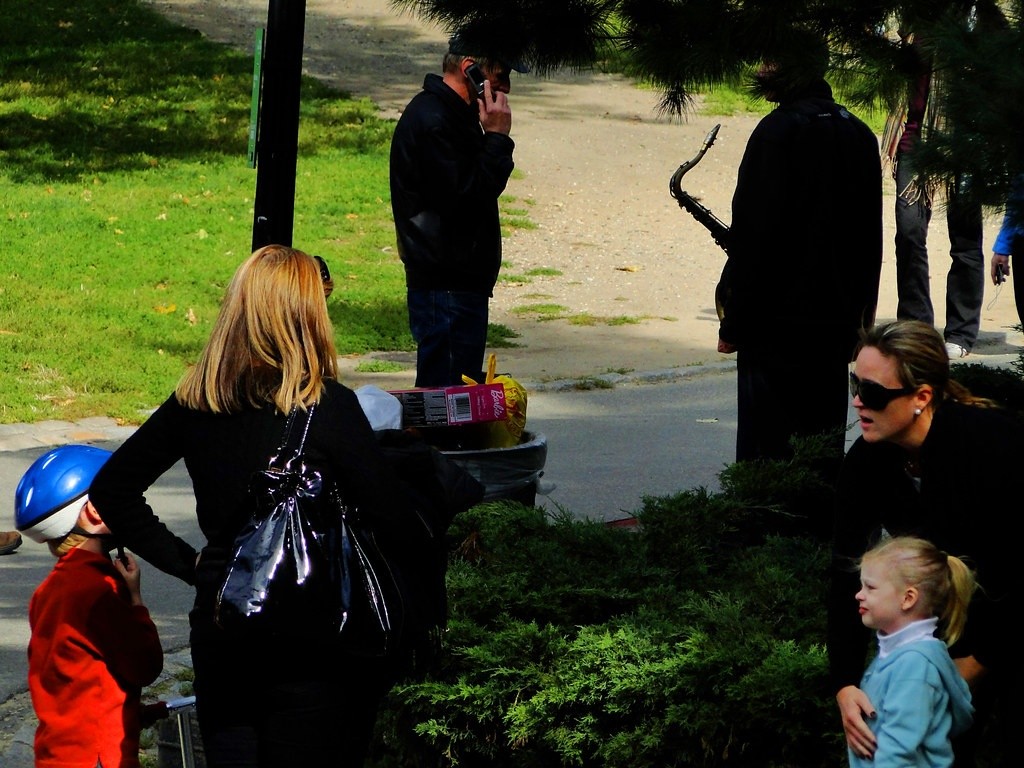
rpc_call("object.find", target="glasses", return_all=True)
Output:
[849,372,916,412]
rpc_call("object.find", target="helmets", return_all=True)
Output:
[15,444,114,542]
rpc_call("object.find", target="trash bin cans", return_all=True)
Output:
[434,425,547,516]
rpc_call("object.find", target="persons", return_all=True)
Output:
[389,18,531,387]
[714,29,883,465]
[88,245,394,768]
[823,21,1024,768]
[11,444,163,768]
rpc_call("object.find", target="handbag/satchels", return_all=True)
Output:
[213,377,390,662]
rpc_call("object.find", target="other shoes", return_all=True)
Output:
[0,532,21,554]
[944,341,968,357]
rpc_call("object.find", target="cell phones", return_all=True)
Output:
[465,63,496,108]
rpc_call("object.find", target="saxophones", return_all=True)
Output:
[669,124,729,258]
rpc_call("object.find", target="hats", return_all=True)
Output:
[448,18,529,75]
